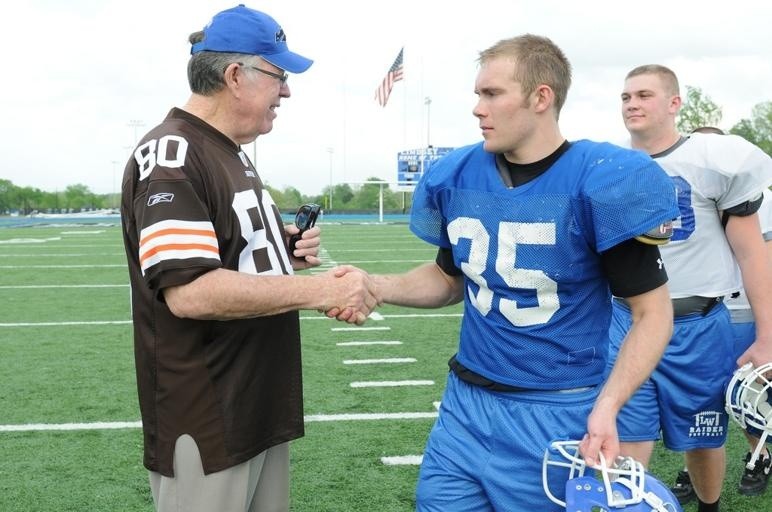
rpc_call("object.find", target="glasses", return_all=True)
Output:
[251,66,289,88]
[289,203,321,261]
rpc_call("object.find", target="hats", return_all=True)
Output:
[191,4,315,74]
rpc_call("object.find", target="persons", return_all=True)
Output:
[599,64,771,512]
[670,127,771,504]
[314,32,680,512]
[117,0,385,512]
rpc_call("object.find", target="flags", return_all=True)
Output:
[372,48,405,109]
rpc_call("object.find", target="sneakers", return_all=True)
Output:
[671,469,697,506]
[737,447,772,495]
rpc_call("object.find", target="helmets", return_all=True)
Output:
[724,363,771,469]
[542,440,683,511]
